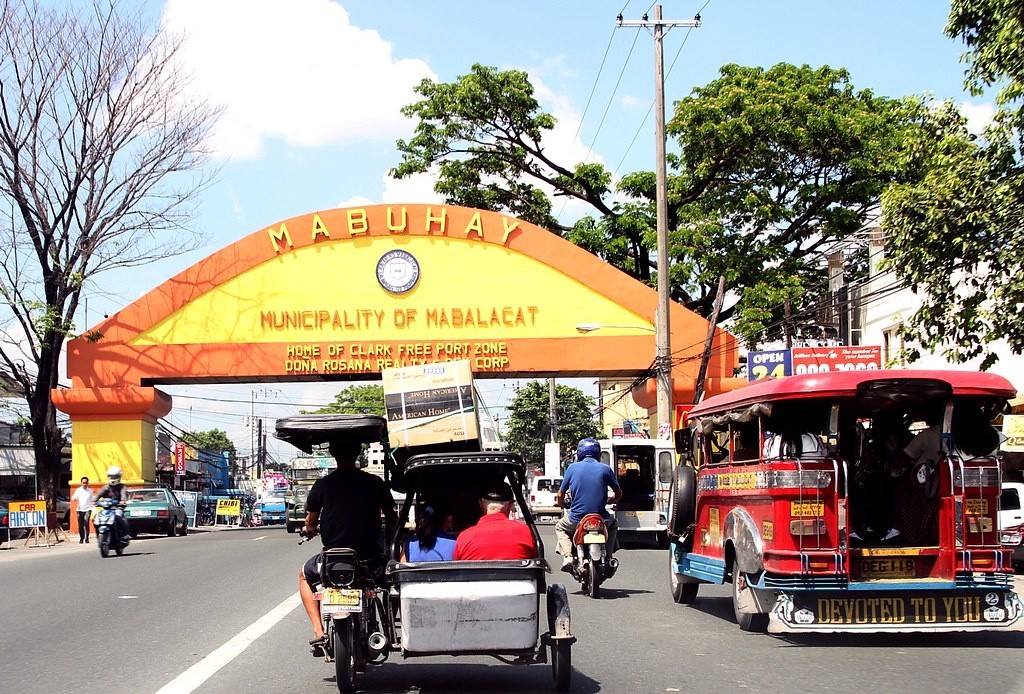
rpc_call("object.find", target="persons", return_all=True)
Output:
[92,466,131,543]
[300,432,398,656]
[555,438,624,573]
[400,480,538,563]
[72,477,95,544]
[243,499,256,527]
[722,398,1001,550]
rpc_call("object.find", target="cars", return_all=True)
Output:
[0,505,25,545]
[124,488,188,539]
[17,486,71,525]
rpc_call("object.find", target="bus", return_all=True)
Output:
[660,369,1024,633]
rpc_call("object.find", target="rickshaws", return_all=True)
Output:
[273,415,577,694]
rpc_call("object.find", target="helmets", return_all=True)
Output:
[577,438,601,460]
[107,466,123,486]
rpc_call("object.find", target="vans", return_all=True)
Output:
[596,438,676,549]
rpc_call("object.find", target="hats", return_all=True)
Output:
[482,481,516,500]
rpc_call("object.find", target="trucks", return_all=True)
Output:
[258,488,288,526]
[284,446,361,533]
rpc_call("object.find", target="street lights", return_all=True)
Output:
[575,324,673,440]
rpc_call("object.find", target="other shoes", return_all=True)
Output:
[561,556,574,569]
[85,539,89,543]
[80,539,83,543]
[863,525,875,533]
[121,535,133,539]
[848,531,864,540]
[313,642,325,657]
[881,528,901,541]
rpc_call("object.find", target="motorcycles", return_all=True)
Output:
[90,498,129,558]
[553,496,619,597]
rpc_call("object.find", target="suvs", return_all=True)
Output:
[526,475,568,521]
